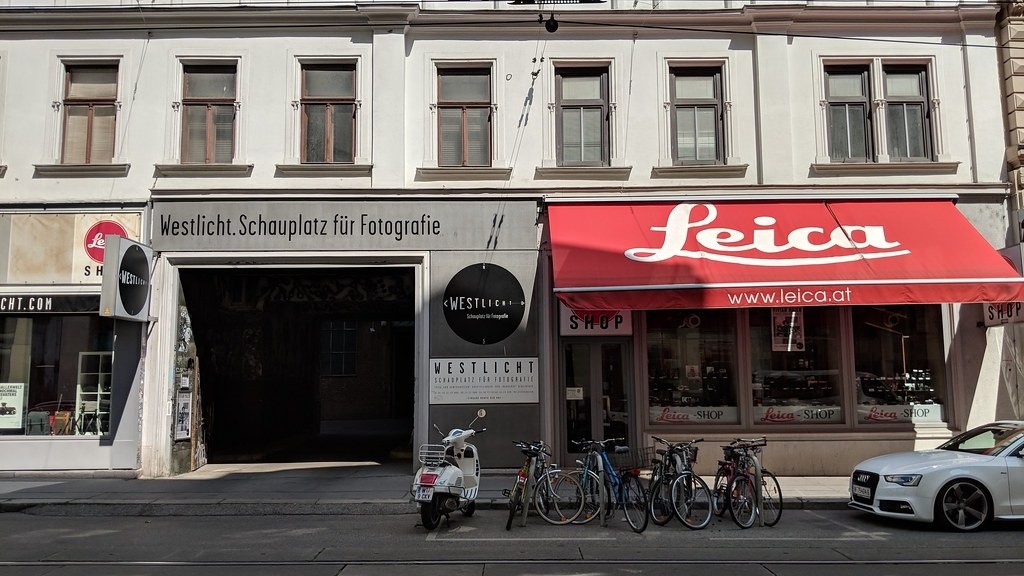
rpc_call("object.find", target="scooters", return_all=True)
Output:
[409,408,487,531]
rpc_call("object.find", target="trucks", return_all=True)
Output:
[753,370,898,406]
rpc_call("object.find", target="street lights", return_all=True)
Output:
[902,335,909,380]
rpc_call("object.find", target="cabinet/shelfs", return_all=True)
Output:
[72,351,112,434]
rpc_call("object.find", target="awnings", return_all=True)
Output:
[547,200,1024,311]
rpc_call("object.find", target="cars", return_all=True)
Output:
[23,400,110,433]
[848,420,1024,533]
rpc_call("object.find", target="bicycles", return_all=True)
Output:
[502,436,784,533]
